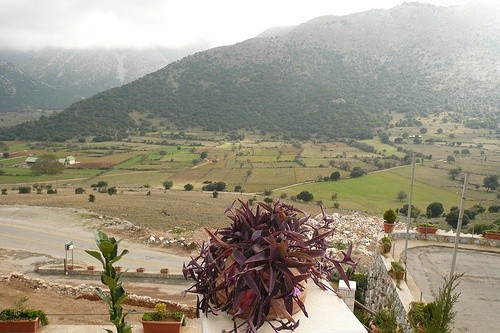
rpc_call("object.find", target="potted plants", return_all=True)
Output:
[382,208,397,233]
[390,260,406,280]
[180,201,361,333]
[484,230,500,239]
[408,272,465,333]
[416,221,439,235]
[0,306,49,333]
[377,237,391,253]
[141,311,185,333]
